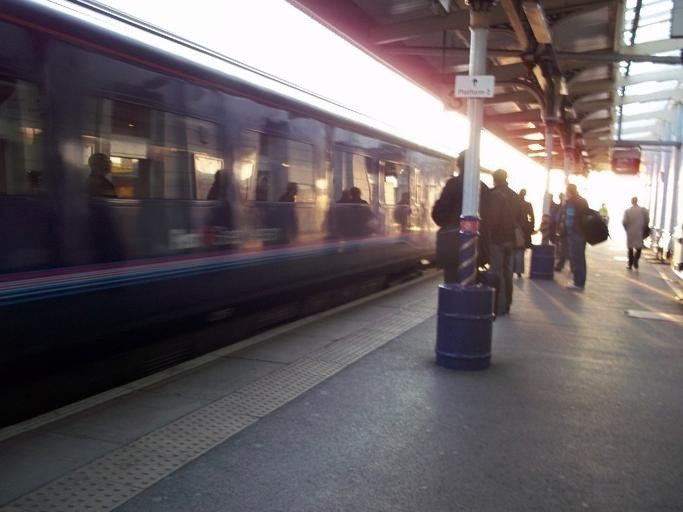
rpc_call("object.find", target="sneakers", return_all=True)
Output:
[565,282,584,290]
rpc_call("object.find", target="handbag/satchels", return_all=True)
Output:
[434,221,457,268]
[643,225,650,238]
[582,208,608,245]
[514,224,529,248]
[478,267,507,314]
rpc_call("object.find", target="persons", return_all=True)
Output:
[207,169,238,252]
[431,150,489,285]
[539,184,609,292]
[71,153,126,264]
[518,188,535,277]
[328,188,379,242]
[622,197,650,269]
[394,192,412,235]
[276,181,298,245]
[489,169,522,316]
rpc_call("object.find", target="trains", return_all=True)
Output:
[0,2,494,429]
